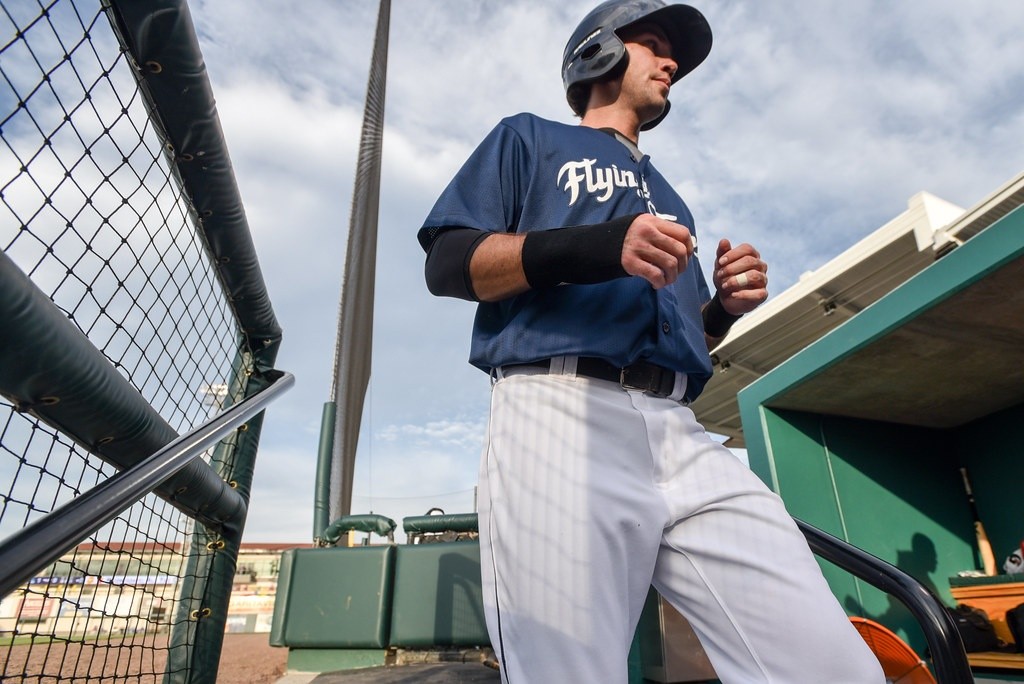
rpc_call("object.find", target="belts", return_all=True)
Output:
[493,355,696,404]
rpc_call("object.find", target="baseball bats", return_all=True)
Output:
[960,468,999,576]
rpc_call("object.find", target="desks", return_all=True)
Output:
[950,577,1024,670]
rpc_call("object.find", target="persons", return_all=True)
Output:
[417,0,887,684]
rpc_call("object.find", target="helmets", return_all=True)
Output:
[561,0,713,132]
